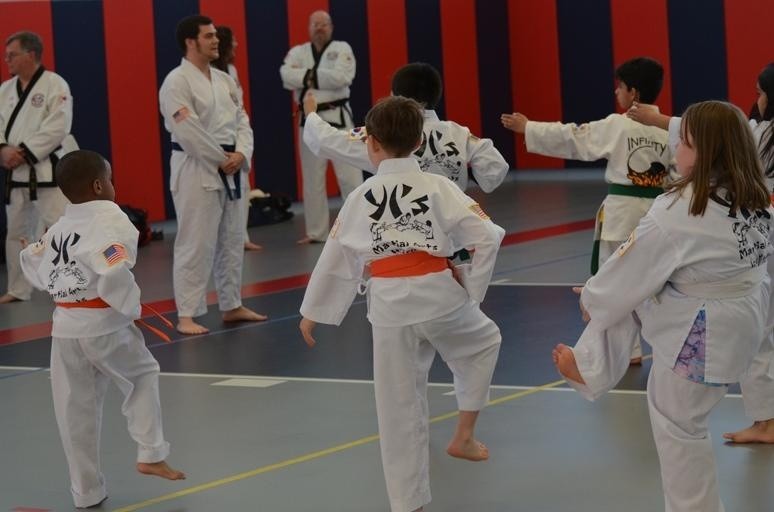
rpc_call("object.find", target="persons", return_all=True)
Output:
[292,62,509,203]
[627,62,772,445]
[499,56,674,369]
[16,147,187,511]
[0,32,81,307]
[547,100,772,511]
[275,10,365,245]
[215,25,270,252]
[296,96,508,511]
[155,14,268,334]
